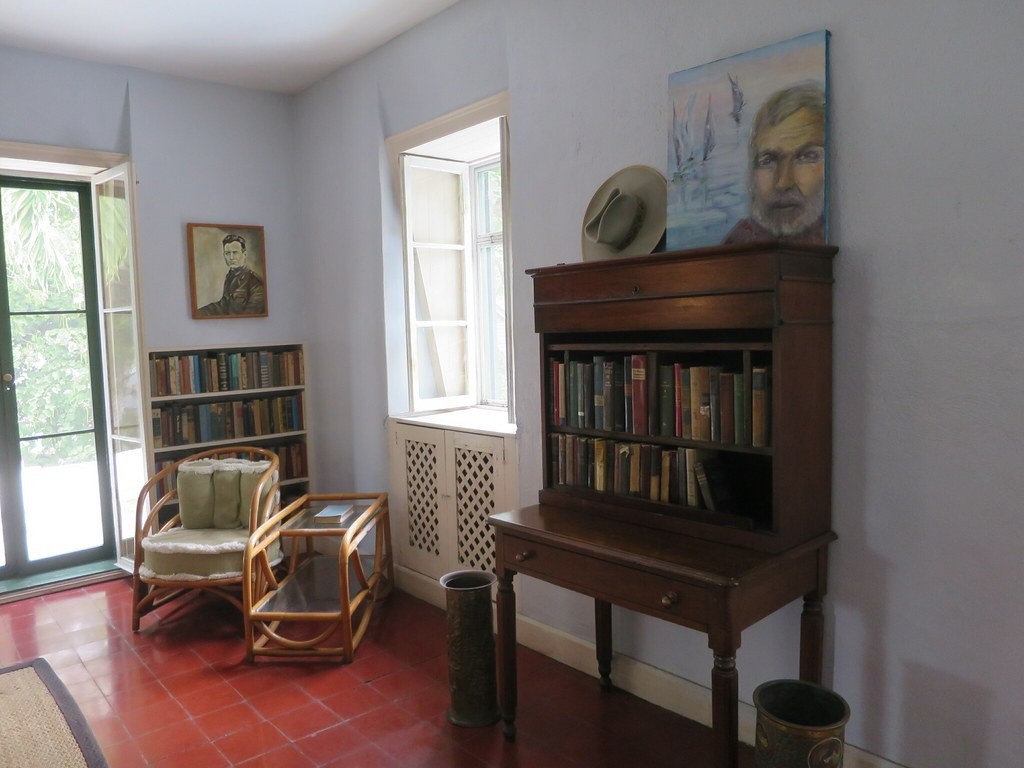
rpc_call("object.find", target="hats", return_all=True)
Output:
[581,164,668,262]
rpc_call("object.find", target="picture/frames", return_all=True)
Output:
[187,222,269,320]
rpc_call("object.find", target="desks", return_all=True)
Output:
[242,490,387,662]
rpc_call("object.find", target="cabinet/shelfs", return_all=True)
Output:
[146,342,313,559]
[488,233,832,768]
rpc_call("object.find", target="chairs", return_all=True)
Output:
[131,445,290,635]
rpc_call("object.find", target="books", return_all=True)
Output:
[148,349,308,529]
[313,504,354,524]
[546,356,770,512]
